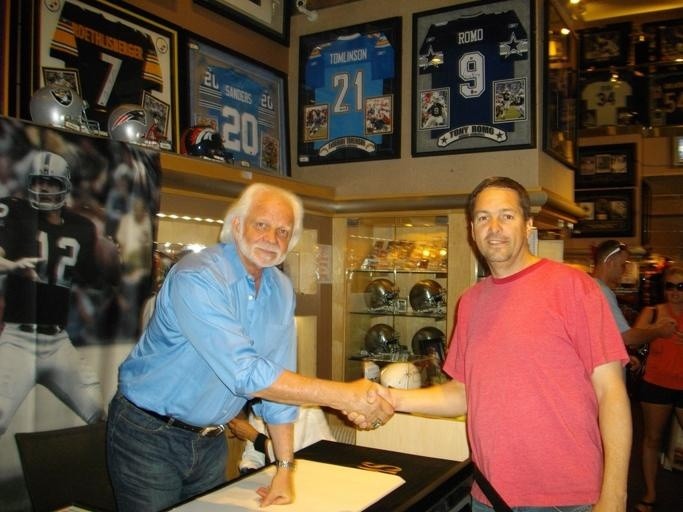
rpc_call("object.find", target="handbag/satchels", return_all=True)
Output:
[625,343,650,377]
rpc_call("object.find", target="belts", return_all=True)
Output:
[17,324,65,335]
[122,396,225,437]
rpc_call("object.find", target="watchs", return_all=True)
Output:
[275,458,295,472]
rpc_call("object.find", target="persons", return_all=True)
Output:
[104,182,394,512]
[0,117,158,344]
[227,397,338,477]
[343,175,633,512]
[366,97,391,133]
[307,108,327,137]
[421,90,447,127]
[495,82,525,120]
[0,153,109,435]
[585,240,683,512]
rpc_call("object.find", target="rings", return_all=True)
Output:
[372,418,384,429]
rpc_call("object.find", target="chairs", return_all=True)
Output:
[14,424,121,512]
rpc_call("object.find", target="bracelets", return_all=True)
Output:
[254,433,268,454]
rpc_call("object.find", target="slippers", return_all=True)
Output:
[639,499,655,512]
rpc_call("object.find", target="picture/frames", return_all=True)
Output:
[411,0,536,157]
[179,28,290,177]
[575,143,637,190]
[193,0,290,47]
[292,0,357,15]
[10,0,181,154]
[573,190,636,238]
[298,15,401,166]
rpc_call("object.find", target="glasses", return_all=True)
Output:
[665,282,683,292]
[604,241,626,263]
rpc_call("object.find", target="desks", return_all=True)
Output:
[160,440,472,512]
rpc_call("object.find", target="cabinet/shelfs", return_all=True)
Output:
[332,209,474,381]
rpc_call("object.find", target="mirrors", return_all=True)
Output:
[543,1,577,170]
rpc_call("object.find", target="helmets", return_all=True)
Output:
[411,326,446,359]
[364,362,380,384]
[26,153,70,209]
[364,278,399,311]
[364,324,402,356]
[381,361,421,391]
[409,279,445,313]
[30,86,89,130]
[180,126,228,159]
[109,105,160,147]
